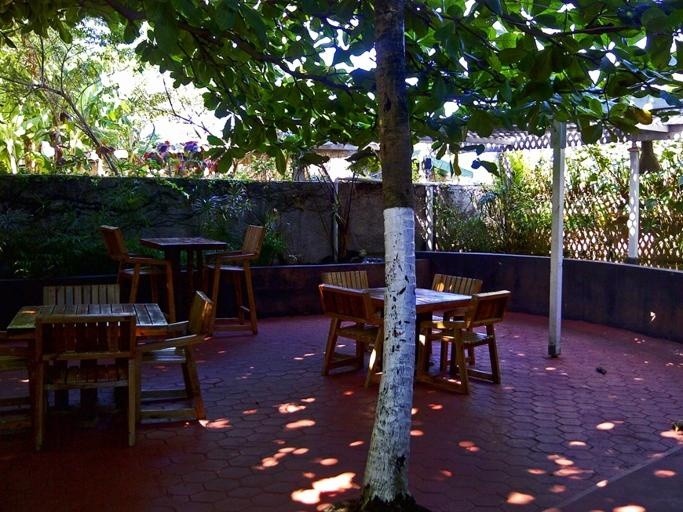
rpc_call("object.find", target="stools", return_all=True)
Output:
[202,225,264,336]
[100,224,176,333]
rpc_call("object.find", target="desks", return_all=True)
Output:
[6,302,168,407]
[138,237,229,320]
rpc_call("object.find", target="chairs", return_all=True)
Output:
[43,285,120,406]
[318,270,512,395]
[31,312,138,449]
[130,290,216,422]
